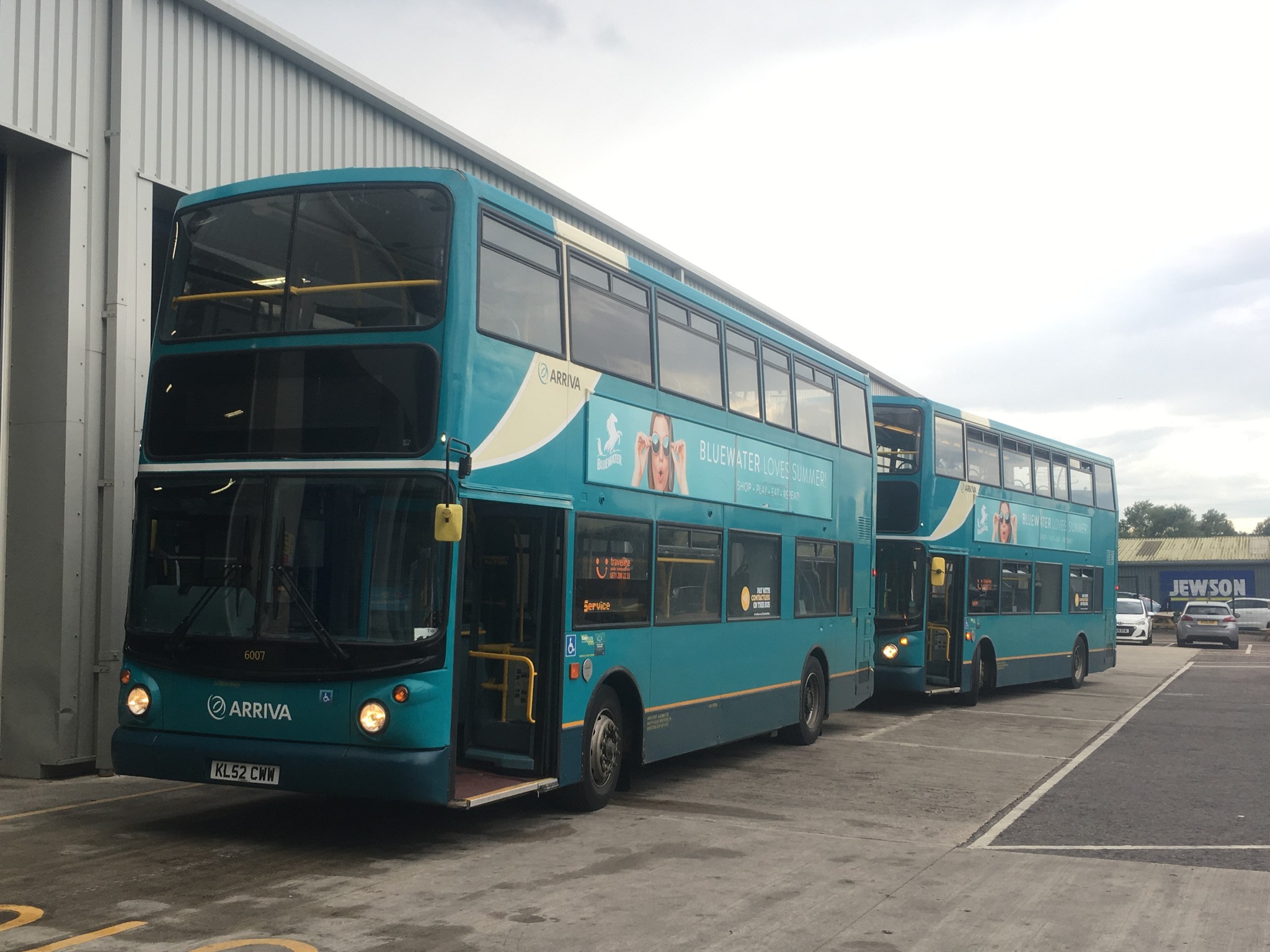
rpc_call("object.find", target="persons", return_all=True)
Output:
[991,499,1022,546]
[631,412,690,497]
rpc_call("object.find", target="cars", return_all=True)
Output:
[1116,591,1161,646]
[1225,597,1270,632]
[1173,601,1240,650]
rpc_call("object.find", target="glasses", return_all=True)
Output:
[650,433,670,457]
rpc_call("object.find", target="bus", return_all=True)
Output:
[109,168,879,816]
[870,393,1116,706]
